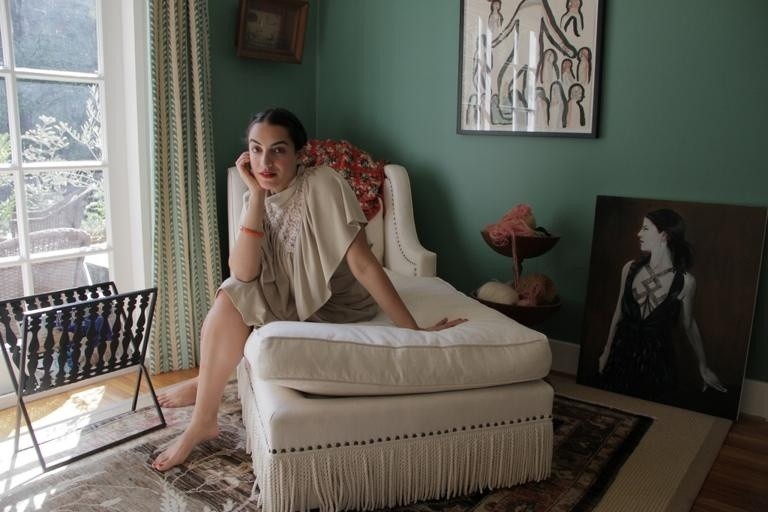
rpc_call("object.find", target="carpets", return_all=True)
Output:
[0,375,732,512]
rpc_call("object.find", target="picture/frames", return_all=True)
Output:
[236,1,309,66]
[456,0,603,141]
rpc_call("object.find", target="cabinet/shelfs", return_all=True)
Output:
[479,224,562,337]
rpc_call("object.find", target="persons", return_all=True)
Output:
[152,108,468,473]
[599,209,728,407]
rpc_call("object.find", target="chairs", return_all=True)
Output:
[1,229,93,346]
[12,185,95,238]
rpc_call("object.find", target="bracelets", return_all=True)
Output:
[238,224,264,239]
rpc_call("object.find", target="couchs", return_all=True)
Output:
[223,165,556,510]
